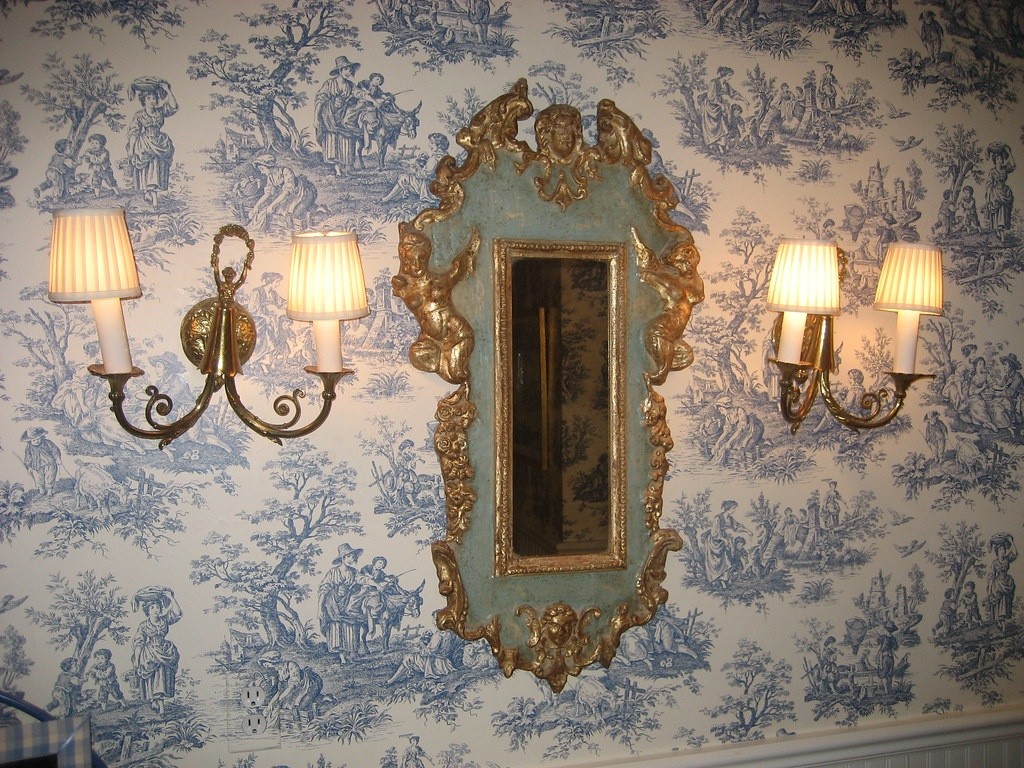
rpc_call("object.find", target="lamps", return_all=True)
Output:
[771,239,943,438]
[49,207,370,450]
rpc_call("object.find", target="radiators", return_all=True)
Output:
[563,695,1024,768]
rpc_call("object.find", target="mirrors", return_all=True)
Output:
[492,237,630,578]
